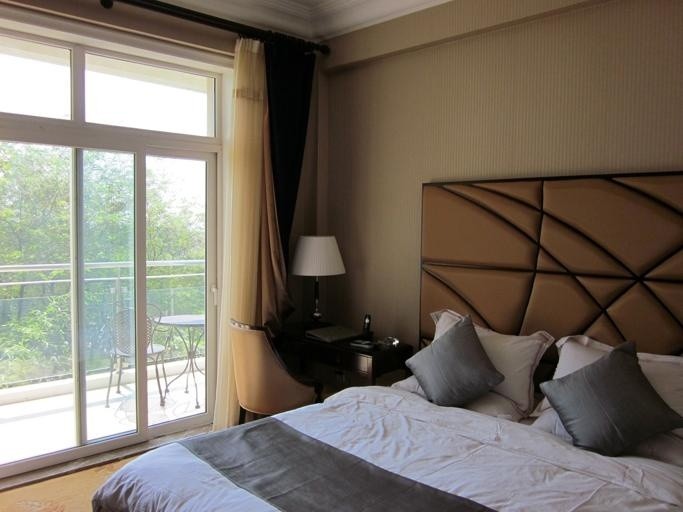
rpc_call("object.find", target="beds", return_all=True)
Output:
[109,169,681,511]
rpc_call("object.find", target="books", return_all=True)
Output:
[305,325,358,343]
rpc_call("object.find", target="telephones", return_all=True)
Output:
[353,314,373,345]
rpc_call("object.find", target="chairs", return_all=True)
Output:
[105,299,170,408]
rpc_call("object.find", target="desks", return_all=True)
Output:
[153,312,209,408]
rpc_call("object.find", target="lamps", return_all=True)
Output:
[289,232,347,327]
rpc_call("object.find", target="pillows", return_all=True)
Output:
[528,335,683,456]
[405,309,555,420]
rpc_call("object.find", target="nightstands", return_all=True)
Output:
[302,336,415,403]
